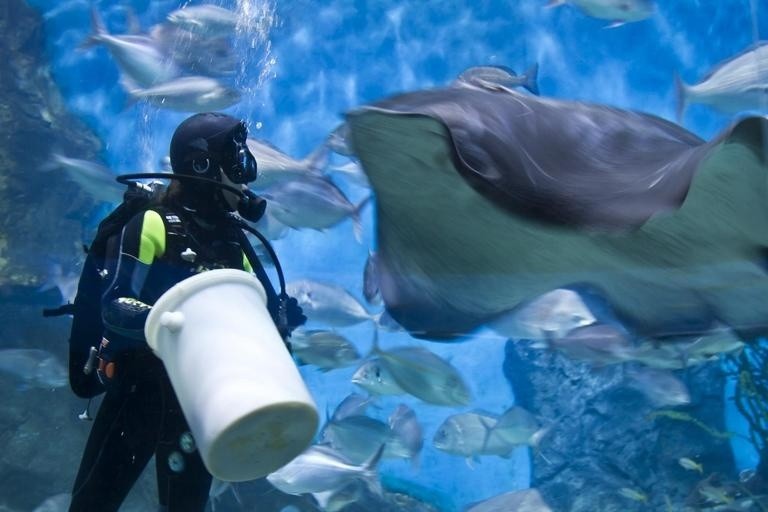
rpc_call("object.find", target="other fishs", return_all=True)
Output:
[1,0,768,511]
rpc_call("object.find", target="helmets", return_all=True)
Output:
[170,114,242,182]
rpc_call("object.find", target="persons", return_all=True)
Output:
[65,109,311,510]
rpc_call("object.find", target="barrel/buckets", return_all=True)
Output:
[143,267,319,481]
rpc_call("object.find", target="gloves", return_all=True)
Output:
[269,292,305,335]
